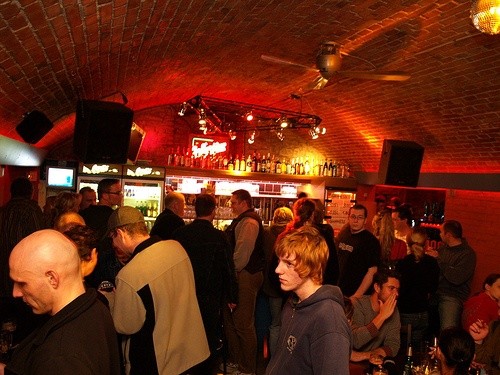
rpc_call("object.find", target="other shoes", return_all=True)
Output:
[226,371,255,375]
[219,362,239,373]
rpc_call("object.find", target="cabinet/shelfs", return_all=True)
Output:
[406,186,446,250]
[77,166,166,233]
[167,167,358,231]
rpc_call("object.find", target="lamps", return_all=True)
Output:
[178,96,327,144]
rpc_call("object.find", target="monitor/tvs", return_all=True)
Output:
[46,166,76,190]
[124,122,146,166]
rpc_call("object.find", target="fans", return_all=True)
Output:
[260,41,411,90]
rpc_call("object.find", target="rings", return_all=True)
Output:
[434,348,437,355]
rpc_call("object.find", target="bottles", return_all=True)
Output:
[404,347,413,375]
[125,189,135,197]
[147,202,158,217]
[422,341,442,375]
[140,201,144,215]
[167,146,352,178]
[424,201,444,224]
[135,201,140,211]
[143,201,147,216]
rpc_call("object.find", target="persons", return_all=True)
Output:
[350,271,401,362]
[0,175,128,366]
[149,192,185,243]
[185,194,238,375]
[98,206,211,375]
[393,227,440,351]
[224,189,266,375]
[263,192,340,358]
[428,327,476,375]
[0,229,121,375]
[392,206,412,255]
[468,300,500,375]
[335,204,381,301]
[265,227,353,375]
[461,273,500,335]
[372,212,407,268]
[435,220,476,338]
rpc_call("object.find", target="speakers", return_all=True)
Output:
[16,110,54,144]
[378,139,425,188]
[73,99,134,164]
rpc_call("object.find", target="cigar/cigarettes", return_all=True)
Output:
[231,308,233,312]
[434,337,436,347]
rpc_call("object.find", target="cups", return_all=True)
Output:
[366,353,384,375]
[184,205,238,217]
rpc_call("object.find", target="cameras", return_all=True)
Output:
[98,281,113,291]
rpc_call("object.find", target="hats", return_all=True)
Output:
[101,206,145,240]
[54,212,86,233]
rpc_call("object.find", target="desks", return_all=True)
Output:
[408,354,498,375]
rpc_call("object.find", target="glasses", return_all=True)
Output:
[349,215,365,219]
[104,192,122,195]
